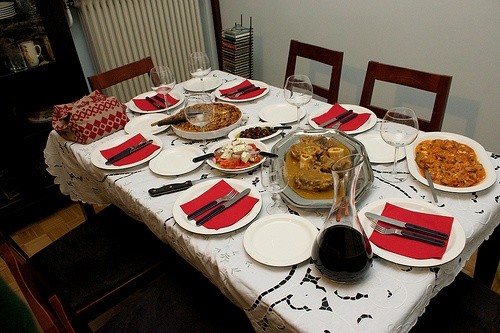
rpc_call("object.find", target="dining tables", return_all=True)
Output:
[44,70,500,333]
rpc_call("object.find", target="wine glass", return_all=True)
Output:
[189,51,211,94]
[380,107,419,183]
[284,74,313,129]
[185,93,215,153]
[261,157,288,215]
[150,66,176,117]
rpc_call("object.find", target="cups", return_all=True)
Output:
[20,41,41,67]
[6,43,27,72]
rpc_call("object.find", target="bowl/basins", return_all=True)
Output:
[170,102,243,140]
[205,138,268,174]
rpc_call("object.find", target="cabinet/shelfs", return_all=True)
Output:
[1,0,92,236]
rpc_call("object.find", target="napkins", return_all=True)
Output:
[367,203,455,260]
[100,133,162,166]
[218,79,266,100]
[311,103,371,131]
[180,179,260,232]
[130,92,180,112]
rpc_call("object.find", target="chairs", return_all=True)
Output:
[1,203,255,333]
[358,60,452,133]
[282,40,344,105]
[87,56,161,104]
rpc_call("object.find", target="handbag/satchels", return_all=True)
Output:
[52,90,130,144]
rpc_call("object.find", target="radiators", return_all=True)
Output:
[65,0,200,101]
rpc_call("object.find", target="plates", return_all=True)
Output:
[275,133,367,205]
[309,103,497,267]
[183,76,270,102]
[229,101,306,141]
[91,89,206,176]
[0,2,17,20]
[172,178,319,267]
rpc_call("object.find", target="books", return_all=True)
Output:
[222,24,253,78]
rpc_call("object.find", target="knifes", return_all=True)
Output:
[148,175,238,197]
[365,212,448,240]
[196,188,251,226]
[146,96,164,109]
[105,140,153,165]
[220,84,254,97]
[318,110,353,127]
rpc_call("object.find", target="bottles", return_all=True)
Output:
[311,154,373,285]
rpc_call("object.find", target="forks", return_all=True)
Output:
[187,189,237,221]
[155,95,171,106]
[333,113,358,129]
[370,222,445,247]
[231,87,260,98]
